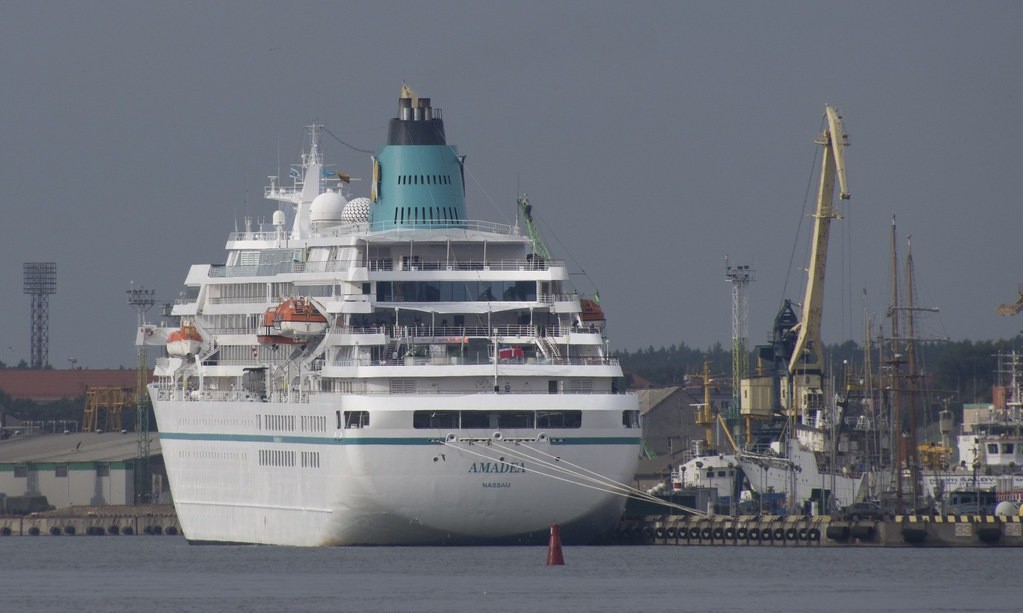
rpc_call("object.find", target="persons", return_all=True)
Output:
[351,316,450,335]
[392,349,399,365]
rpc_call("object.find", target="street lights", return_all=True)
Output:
[126,289,155,504]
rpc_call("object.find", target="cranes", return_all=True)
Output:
[741,104,851,447]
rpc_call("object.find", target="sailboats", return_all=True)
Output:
[673,215,1023,513]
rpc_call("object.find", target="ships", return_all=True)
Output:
[144,84,642,546]
